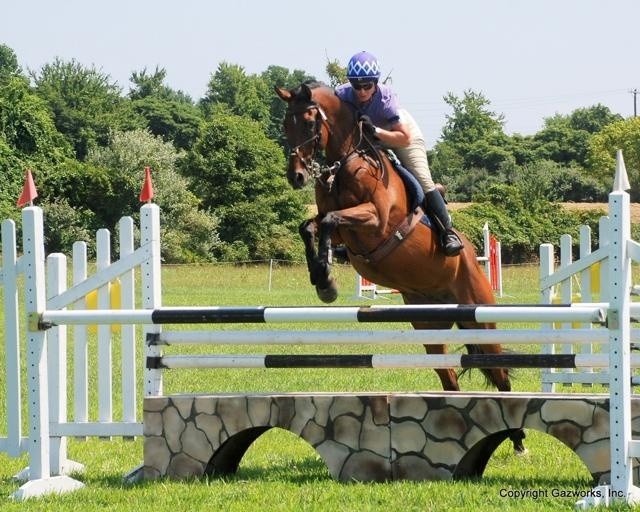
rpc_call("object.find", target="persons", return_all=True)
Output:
[334,50,465,261]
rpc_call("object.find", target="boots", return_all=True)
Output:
[334,247,346,258]
[425,188,463,254]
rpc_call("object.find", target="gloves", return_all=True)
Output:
[360,115,375,136]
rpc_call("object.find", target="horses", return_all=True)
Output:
[274,80,528,457]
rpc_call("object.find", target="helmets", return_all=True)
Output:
[347,51,380,80]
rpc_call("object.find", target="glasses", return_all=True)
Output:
[353,83,373,91]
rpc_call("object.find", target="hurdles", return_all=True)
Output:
[349,220,504,300]
[0,190,640,507]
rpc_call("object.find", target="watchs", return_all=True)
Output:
[374,127,381,137]
